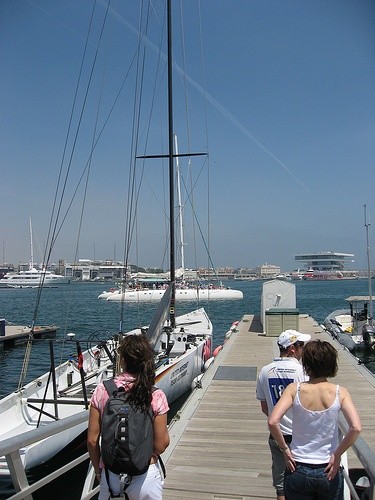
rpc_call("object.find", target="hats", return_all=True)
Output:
[277,329,311,349]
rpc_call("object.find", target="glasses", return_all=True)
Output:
[296,342,304,347]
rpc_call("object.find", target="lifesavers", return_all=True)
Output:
[202,338,211,361]
[203,357,215,370]
[208,284,213,289]
[212,344,223,356]
[224,320,240,339]
[190,373,205,390]
[110,287,115,293]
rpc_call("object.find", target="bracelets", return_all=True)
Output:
[279,445,288,452]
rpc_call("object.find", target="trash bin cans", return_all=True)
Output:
[264,308,300,337]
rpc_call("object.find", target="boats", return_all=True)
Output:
[324,293,373,361]
[97,2,246,300]
[1,0,214,479]
[0,270,71,288]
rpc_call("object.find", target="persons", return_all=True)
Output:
[256,329,311,500]
[129,282,225,291]
[268,339,362,500]
[87,334,169,500]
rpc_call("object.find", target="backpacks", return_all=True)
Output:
[101,378,159,476]
